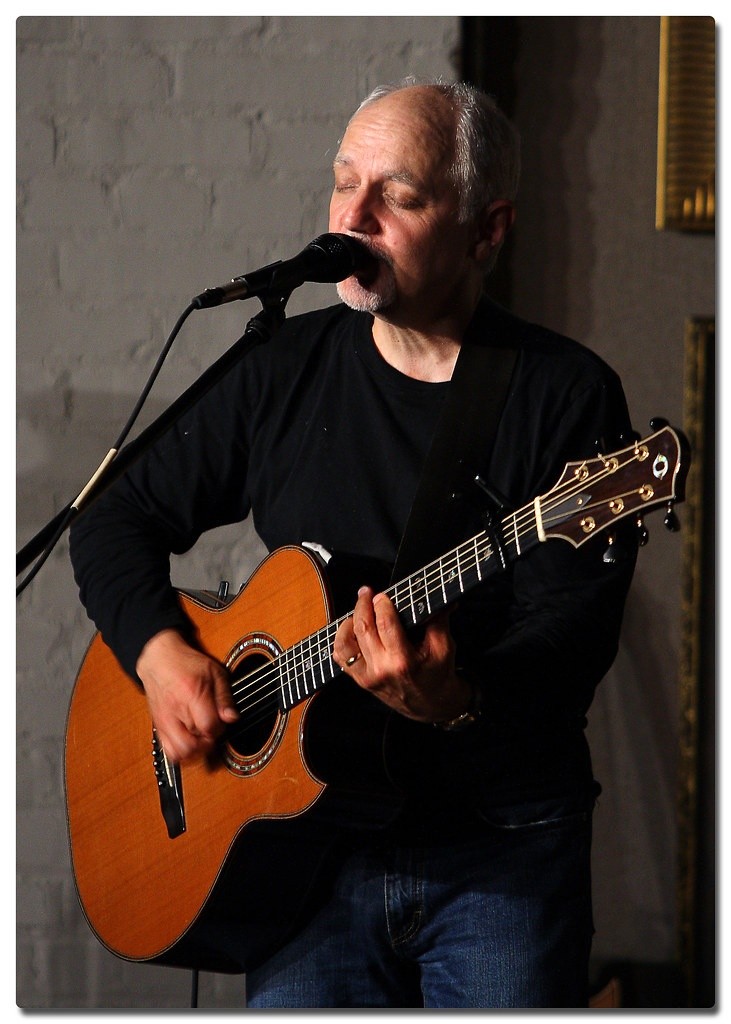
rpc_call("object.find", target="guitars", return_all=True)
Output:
[63,411,695,975]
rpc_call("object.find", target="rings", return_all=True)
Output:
[346,653,362,667]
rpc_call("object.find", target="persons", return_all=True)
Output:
[68,78,639,1008]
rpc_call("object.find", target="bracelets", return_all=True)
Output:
[432,684,482,730]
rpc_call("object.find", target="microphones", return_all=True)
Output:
[192,232,365,310]
